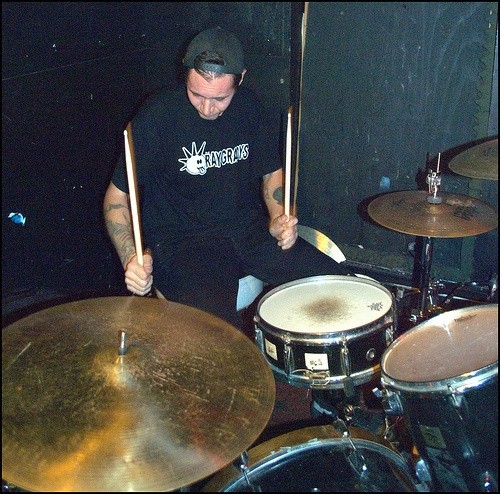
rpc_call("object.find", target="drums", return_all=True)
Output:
[251,272,400,392]
[378,302,499,493]
[198,421,433,494]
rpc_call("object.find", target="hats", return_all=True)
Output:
[184,26,245,75]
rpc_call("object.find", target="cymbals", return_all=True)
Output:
[0,293,278,494]
[366,189,499,239]
[447,136,499,182]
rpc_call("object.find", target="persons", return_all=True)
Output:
[101,23,359,326]
